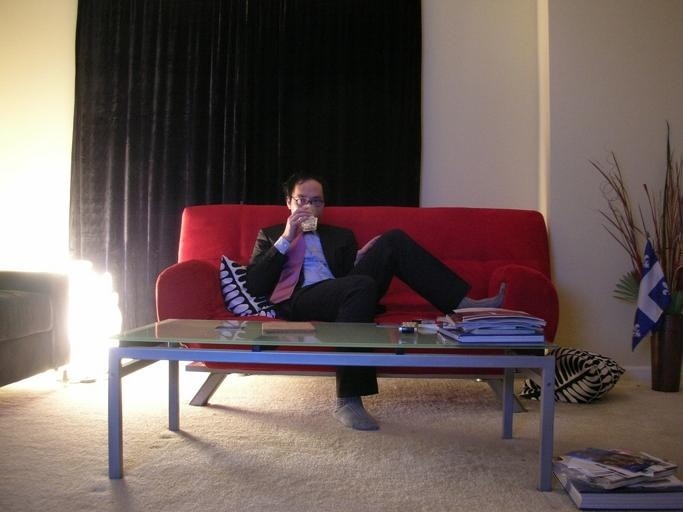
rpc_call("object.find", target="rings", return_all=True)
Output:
[297,219,302,222]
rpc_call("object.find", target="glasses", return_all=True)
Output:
[288,195,324,207]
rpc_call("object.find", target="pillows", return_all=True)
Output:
[219,252,277,318]
[520,345,626,404]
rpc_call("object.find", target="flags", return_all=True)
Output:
[630,238,671,354]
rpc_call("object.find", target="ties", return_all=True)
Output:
[268,226,306,305]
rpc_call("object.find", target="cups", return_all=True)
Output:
[299,205,318,232]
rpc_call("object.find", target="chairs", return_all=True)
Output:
[0,271,71,389]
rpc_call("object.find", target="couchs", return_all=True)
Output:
[154,204,559,412]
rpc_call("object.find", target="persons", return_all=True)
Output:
[243,167,509,432]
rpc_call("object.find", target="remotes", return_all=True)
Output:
[399,321,418,333]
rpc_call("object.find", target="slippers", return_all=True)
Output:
[331,400,380,431]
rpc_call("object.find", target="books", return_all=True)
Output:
[260,320,317,333]
[429,305,550,345]
[546,445,682,511]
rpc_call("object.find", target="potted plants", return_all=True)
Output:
[584,119,683,393]
